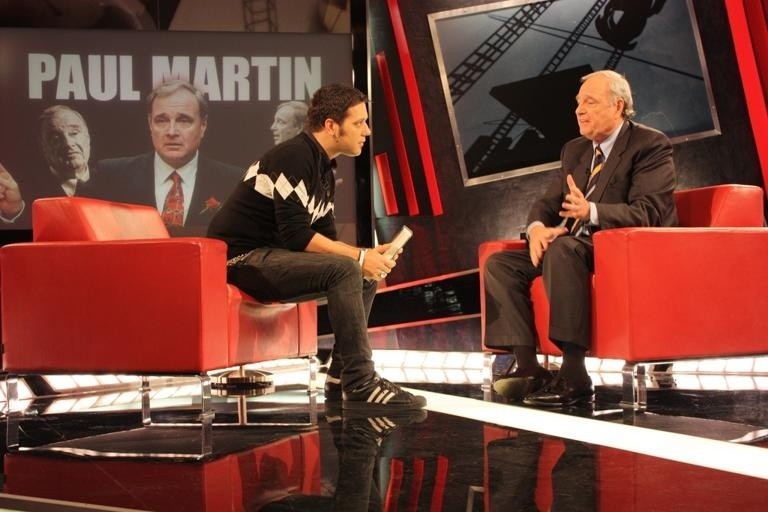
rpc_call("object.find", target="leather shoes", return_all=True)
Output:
[492,366,555,401]
[521,375,594,408]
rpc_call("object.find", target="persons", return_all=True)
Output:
[486,431,601,512]
[0,77,249,233]
[255,410,428,511]
[207,83,428,410]
[270,100,310,147]
[34,104,99,198]
[482,70,678,408]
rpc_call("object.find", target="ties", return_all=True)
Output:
[160,170,185,228]
[567,143,605,237]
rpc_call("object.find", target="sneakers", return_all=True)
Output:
[339,370,427,414]
[321,371,343,403]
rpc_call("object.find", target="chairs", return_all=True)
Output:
[470,177,768,419]
[473,415,768,510]
[0,417,327,511]
[3,191,323,422]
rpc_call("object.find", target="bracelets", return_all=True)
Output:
[356,246,367,272]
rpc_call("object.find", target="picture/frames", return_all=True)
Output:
[425,0,725,189]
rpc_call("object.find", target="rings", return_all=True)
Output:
[379,271,386,279]
